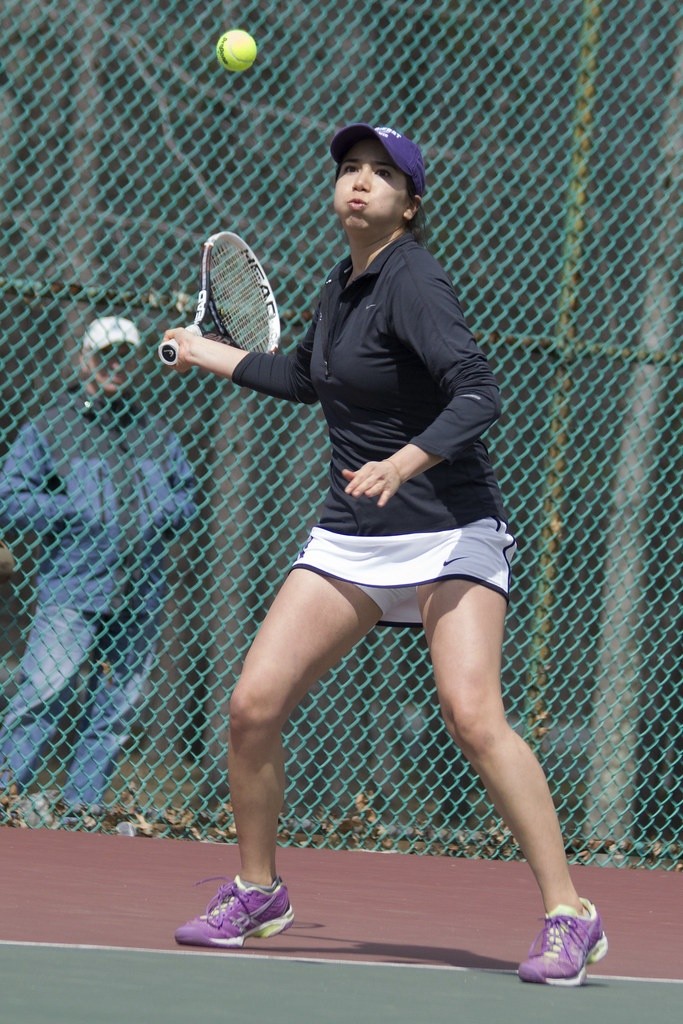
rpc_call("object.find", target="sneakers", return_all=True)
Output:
[519,899,610,987]
[175,875,295,948]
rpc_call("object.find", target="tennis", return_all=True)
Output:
[216,29,258,71]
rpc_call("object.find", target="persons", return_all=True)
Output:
[164,124,607,986]
[1,317,198,825]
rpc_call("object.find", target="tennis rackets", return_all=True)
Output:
[157,231,281,366]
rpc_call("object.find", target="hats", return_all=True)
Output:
[84,315,139,354]
[331,125,425,196]
